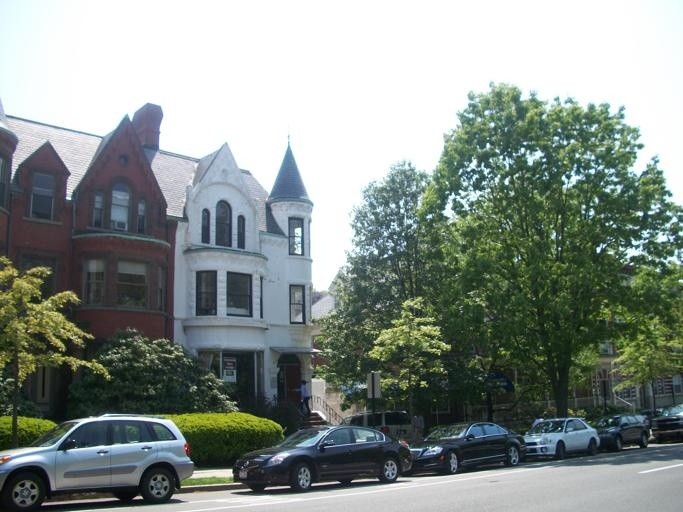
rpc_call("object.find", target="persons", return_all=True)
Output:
[411,412,424,440]
[300,380,310,414]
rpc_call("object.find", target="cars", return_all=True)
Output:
[1,412,194,512]
